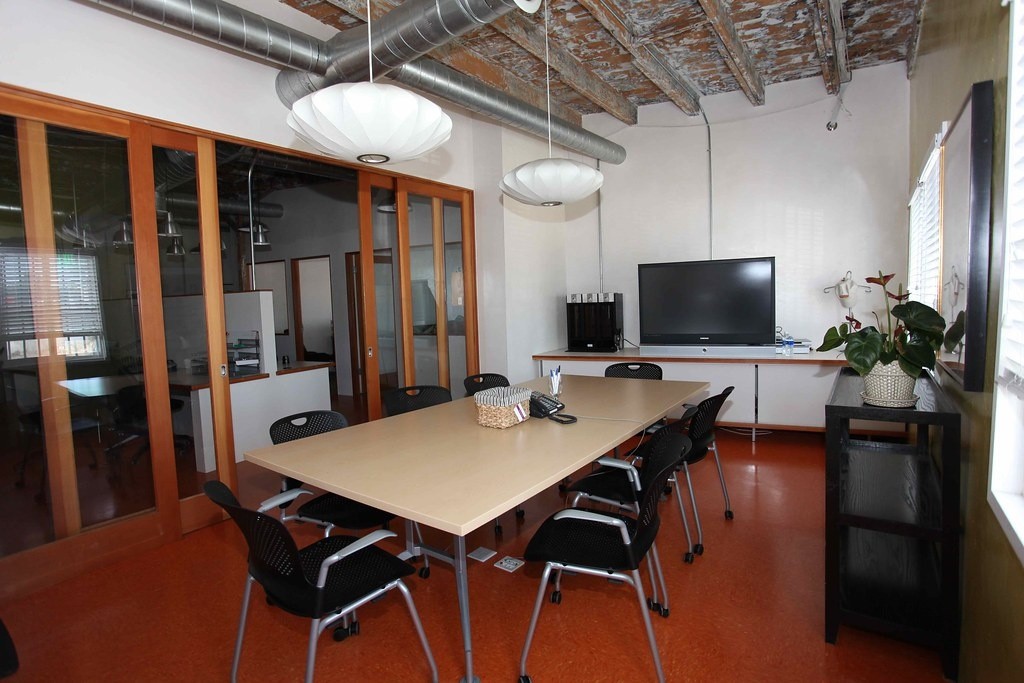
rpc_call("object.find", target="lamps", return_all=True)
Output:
[238,170,270,247]
[498,0,604,206]
[111,219,133,245]
[285,0,452,164]
[189,243,200,255]
[377,194,413,212]
[71,167,97,250]
[158,165,186,256]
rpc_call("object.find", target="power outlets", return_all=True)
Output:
[493,555,525,572]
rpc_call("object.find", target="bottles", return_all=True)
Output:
[191,353,208,375]
[782,332,794,356]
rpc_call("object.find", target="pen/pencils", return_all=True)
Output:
[548,365,561,401]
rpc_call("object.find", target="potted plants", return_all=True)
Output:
[818,269,945,402]
[944,307,966,380]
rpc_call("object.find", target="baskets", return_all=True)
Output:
[473,386,531,429]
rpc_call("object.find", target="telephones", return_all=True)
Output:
[529,390,565,418]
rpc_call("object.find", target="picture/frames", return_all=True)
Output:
[934,79,994,393]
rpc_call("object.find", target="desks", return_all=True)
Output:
[532,346,852,442]
[276,360,338,424]
[54,373,144,483]
[244,372,712,683]
[135,369,269,474]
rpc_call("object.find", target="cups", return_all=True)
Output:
[549,376,562,395]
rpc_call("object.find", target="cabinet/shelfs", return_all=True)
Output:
[825,365,962,682]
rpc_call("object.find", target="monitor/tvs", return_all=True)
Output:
[637,256,776,359]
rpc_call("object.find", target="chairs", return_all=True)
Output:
[392,373,573,579]
[103,385,192,465]
[519,362,735,683]
[203,409,438,683]
[0,369,100,502]
